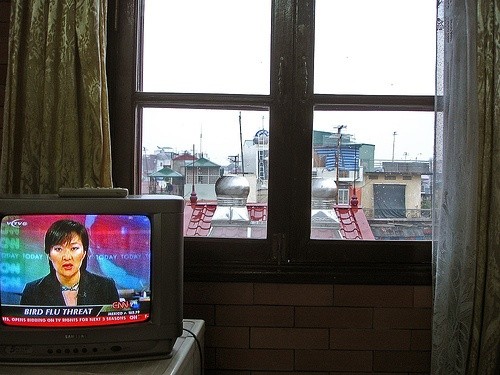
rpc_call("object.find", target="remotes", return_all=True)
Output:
[56,186,130,200]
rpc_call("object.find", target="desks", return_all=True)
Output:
[1,319,206,375]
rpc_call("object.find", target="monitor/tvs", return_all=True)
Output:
[0,192,184,369]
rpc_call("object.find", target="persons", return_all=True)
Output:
[20,219,119,305]
